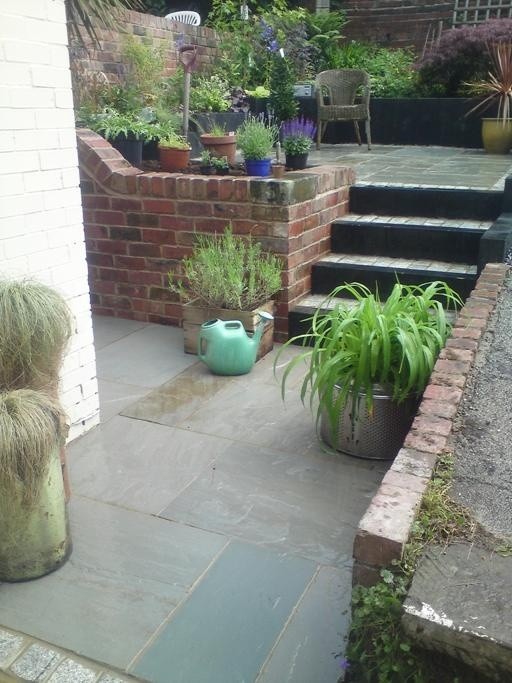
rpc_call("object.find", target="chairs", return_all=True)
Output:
[315,68,372,151]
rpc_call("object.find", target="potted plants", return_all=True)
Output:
[0,276,76,584]
[459,34,512,156]
[166,224,285,365]
[274,268,466,460]
[76,36,317,177]
